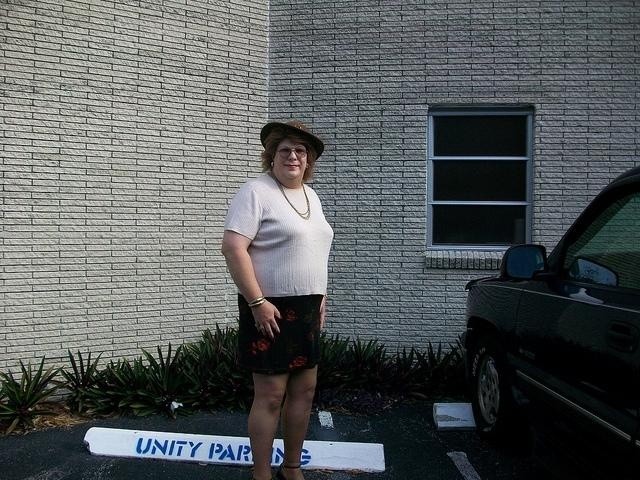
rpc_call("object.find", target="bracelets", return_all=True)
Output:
[247,297,265,308]
[324,293,329,300]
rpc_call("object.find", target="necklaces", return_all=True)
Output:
[272,172,310,220]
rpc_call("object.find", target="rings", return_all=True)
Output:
[258,326,264,330]
[255,324,258,326]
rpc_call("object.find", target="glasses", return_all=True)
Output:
[276,146,307,158]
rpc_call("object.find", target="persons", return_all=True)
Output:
[221,120,335,480]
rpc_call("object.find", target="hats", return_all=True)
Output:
[260,120,324,160]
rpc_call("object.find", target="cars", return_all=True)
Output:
[464,164,639,480]
